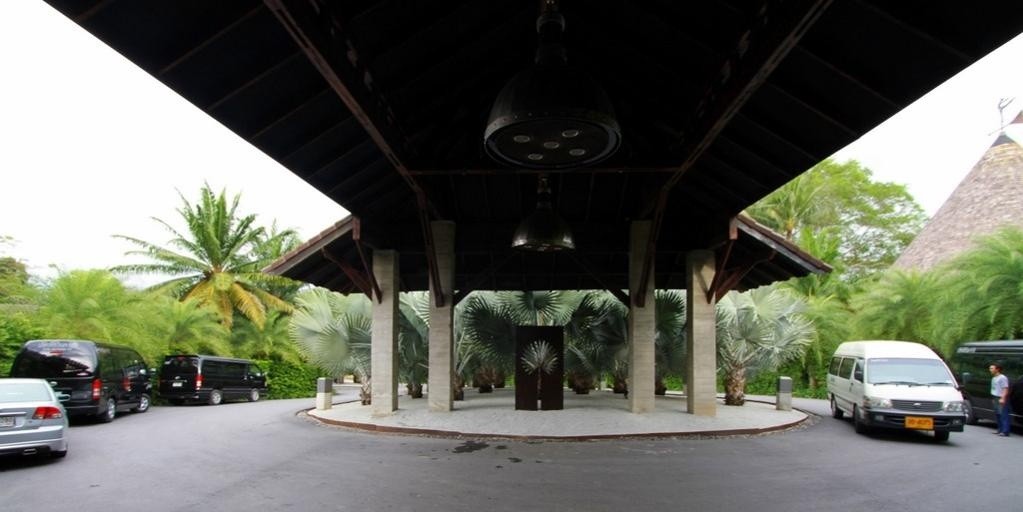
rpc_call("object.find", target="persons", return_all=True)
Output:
[989,363,1010,437]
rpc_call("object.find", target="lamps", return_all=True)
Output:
[480,0,627,257]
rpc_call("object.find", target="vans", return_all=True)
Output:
[948,339,1022,430]
[158,354,268,406]
[825,340,966,440]
[8,339,153,424]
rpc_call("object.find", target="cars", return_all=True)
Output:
[0,377,69,457]
